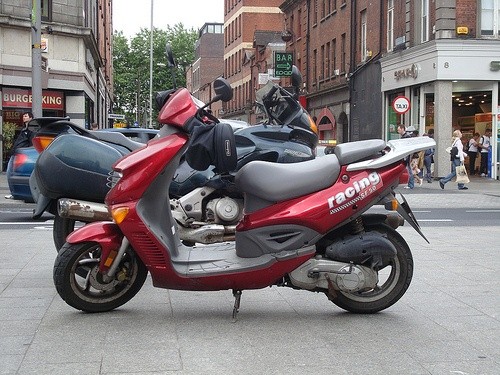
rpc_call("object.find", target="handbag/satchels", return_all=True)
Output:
[455,163,471,184]
[446,147,458,161]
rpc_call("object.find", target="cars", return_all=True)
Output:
[6,116,70,200]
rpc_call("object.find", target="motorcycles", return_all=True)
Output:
[29,66,320,252]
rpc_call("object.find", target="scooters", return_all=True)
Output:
[53,40,437,324]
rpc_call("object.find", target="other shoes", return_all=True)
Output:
[458,187,469,190]
[486,174,488,177]
[404,186,411,189]
[418,179,423,188]
[480,173,486,177]
[440,181,444,189]
[5,195,14,199]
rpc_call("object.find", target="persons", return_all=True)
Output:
[439,130,469,190]
[92,123,98,130]
[468,129,493,177]
[410,129,434,187]
[6,112,34,199]
[397,125,414,189]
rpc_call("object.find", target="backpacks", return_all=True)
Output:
[465,140,473,156]
[478,136,484,152]
